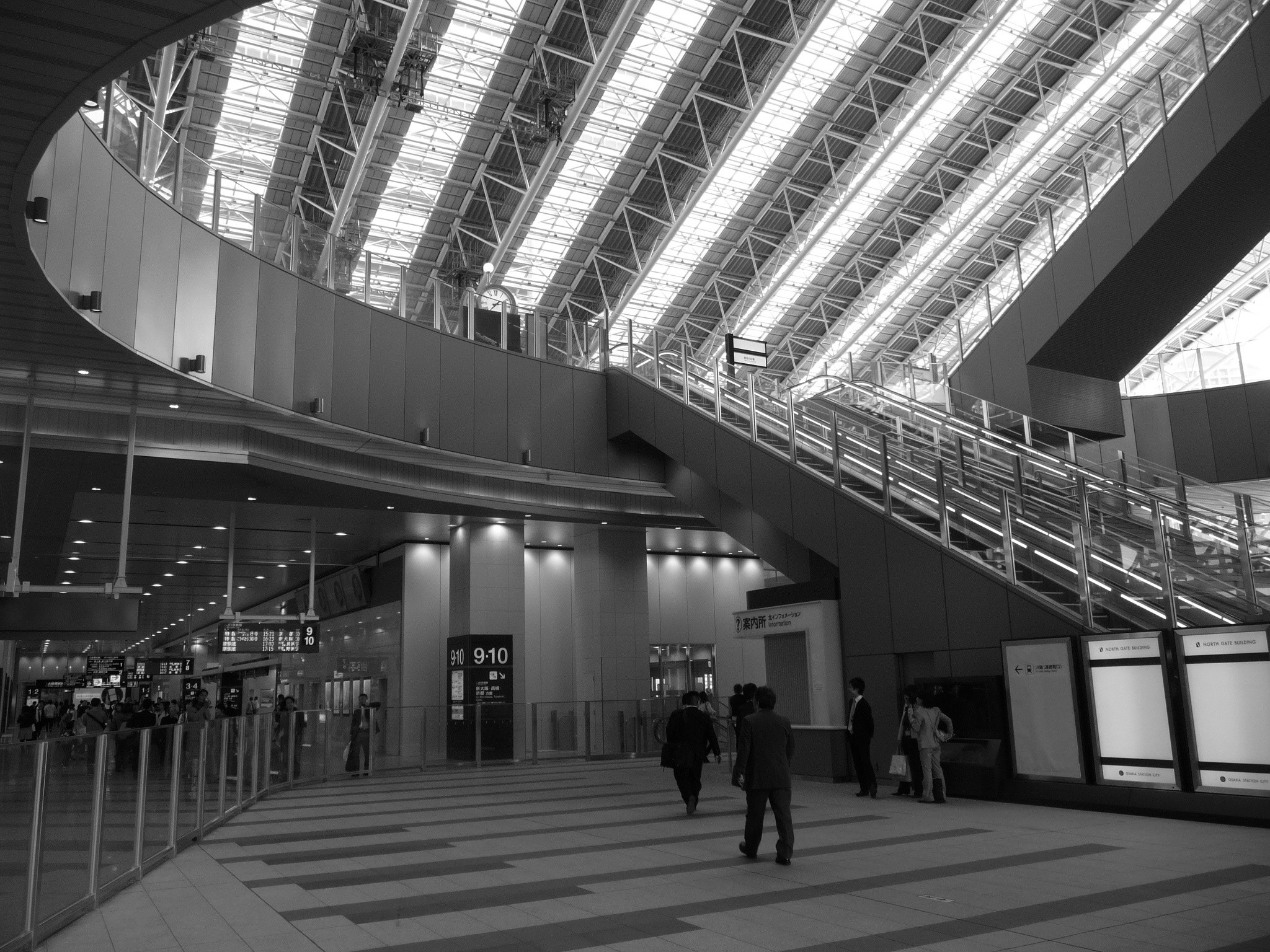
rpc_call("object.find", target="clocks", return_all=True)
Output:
[478,283,518,314]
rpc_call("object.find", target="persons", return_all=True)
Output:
[728,681,796,865]
[844,678,878,798]
[349,694,382,777]
[665,689,721,816]
[890,687,954,803]
[16,689,307,792]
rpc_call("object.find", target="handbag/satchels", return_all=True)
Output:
[705,701,717,720]
[345,742,357,772]
[893,745,912,782]
[936,729,946,742]
[100,722,107,732]
[343,741,351,762]
[888,744,907,777]
[930,778,946,803]
[660,742,695,769]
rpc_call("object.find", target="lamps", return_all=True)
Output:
[82,291,102,313]
[523,449,531,462]
[483,262,494,286]
[420,428,430,443]
[83,90,100,110]
[28,197,49,224]
[310,398,324,414]
[190,355,206,372]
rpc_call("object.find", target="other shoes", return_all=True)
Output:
[775,857,791,866]
[739,842,757,859]
[870,784,877,798]
[686,795,696,814]
[704,756,710,763]
[363,773,369,776]
[855,791,869,797]
[917,798,935,803]
[351,774,360,777]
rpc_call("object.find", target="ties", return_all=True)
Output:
[848,699,856,731]
[362,707,367,730]
[37,709,41,723]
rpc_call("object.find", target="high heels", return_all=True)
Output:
[892,792,908,796]
